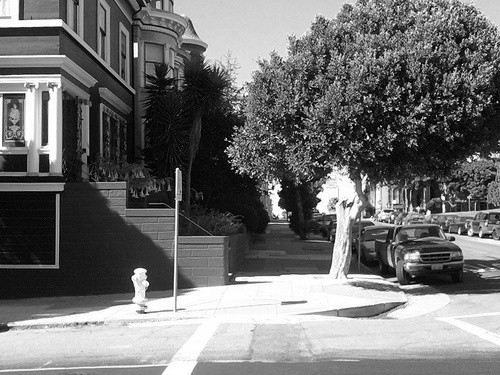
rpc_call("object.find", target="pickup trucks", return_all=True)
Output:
[374,224,465,285]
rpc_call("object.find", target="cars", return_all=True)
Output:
[352,220,375,252]
[430,215,440,224]
[307,206,337,243]
[468,211,500,240]
[355,224,402,267]
[379,208,428,223]
[446,216,475,235]
[434,214,460,232]
